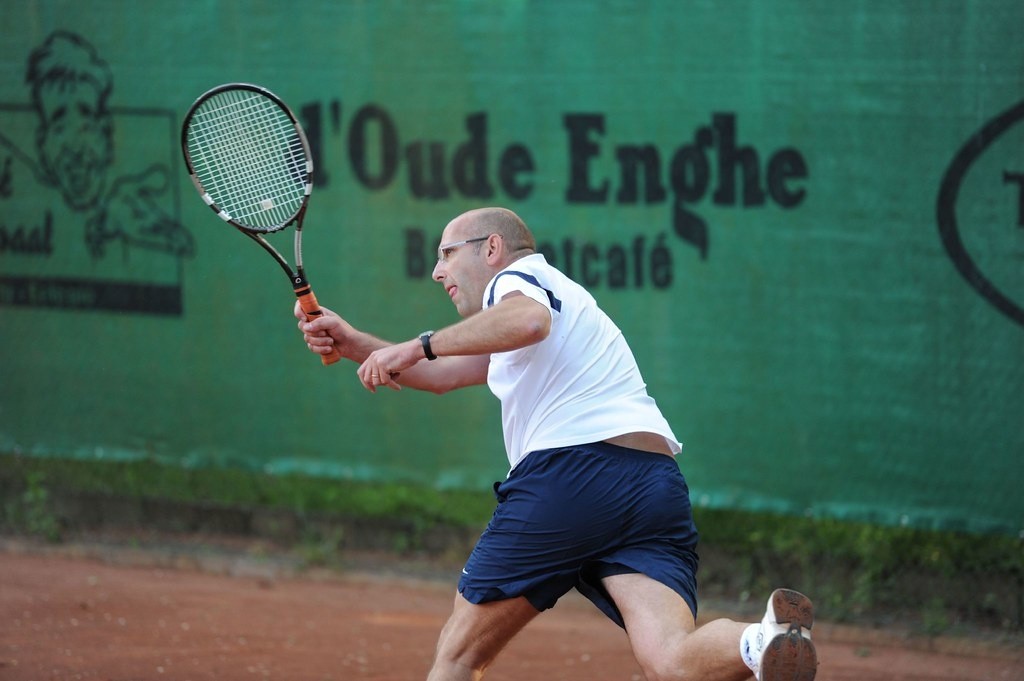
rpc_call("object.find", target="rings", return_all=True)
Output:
[371,374,380,378]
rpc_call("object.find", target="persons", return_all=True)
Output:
[294,208,817,681]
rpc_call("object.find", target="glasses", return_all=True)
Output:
[433,234,503,261]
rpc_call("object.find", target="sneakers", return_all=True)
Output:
[756,587,817,681]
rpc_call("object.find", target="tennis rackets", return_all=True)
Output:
[181,83,340,366]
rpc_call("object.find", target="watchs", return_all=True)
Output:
[419,330,437,361]
